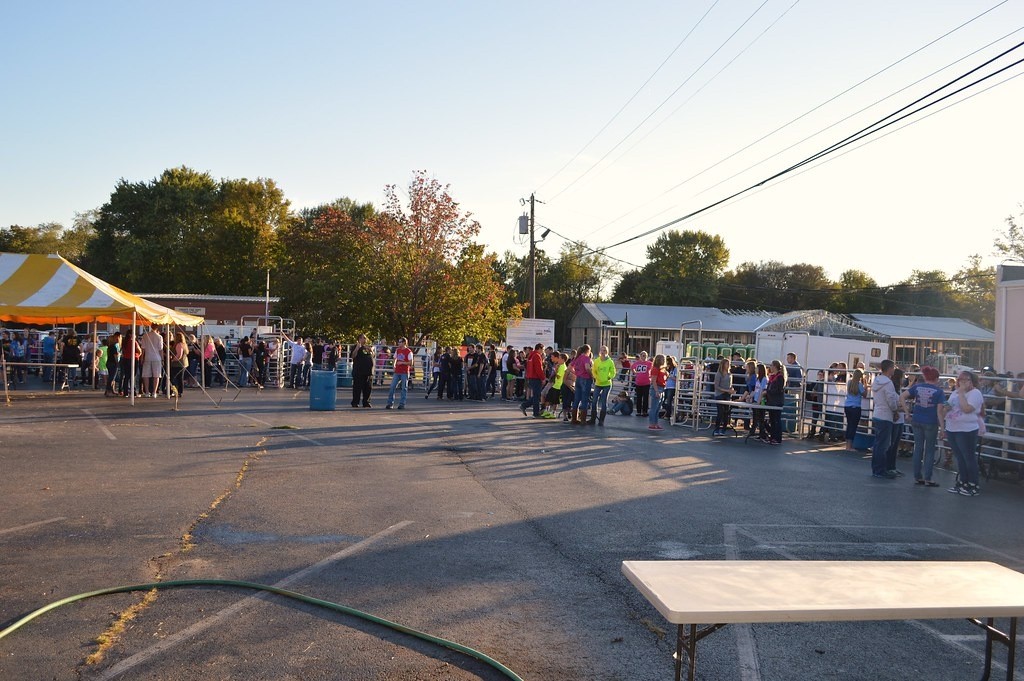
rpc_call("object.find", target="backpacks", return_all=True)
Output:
[16,345,25,357]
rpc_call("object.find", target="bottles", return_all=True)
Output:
[761,395,766,406]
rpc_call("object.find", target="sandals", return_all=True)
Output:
[924,482,940,487]
[915,478,925,484]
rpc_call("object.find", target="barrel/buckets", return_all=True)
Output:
[337,363,352,386]
[310,368,337,410]
[782,394,799,432]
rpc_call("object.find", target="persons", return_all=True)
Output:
[0,322,616,426]
[608,350,1024,480]
[349,333,374,408]
[900,366,947,487]
[386,337,413,409]
[943,371,983,496]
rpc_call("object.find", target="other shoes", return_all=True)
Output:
[714,431,718,435]
[745,432,782,445]
[643,414,647,416]
[636,413,641,416]
[720,431,726,435]
[425,394,521,403]
[517,400,606,427]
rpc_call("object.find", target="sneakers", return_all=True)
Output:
[875,471,896,479]
[648,424,664,430]
[145,392,150,398]
[889,469,906,476]
[386,404,394,409]
[607,409,615,415]
[152,392,157,397]
[365,403,372,408]
[352,403,359,407]
[398,405,405,409]
[947,481,970,493]
[959,483,980,496]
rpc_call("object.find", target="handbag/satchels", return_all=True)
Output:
[172,358,184,367]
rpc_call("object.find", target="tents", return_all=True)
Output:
[0,253,204,405]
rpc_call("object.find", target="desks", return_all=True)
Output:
[697,398,784,444]
[0,362,80,390]
[623,562,1024,681]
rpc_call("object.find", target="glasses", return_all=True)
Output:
[399,340,404,343]
